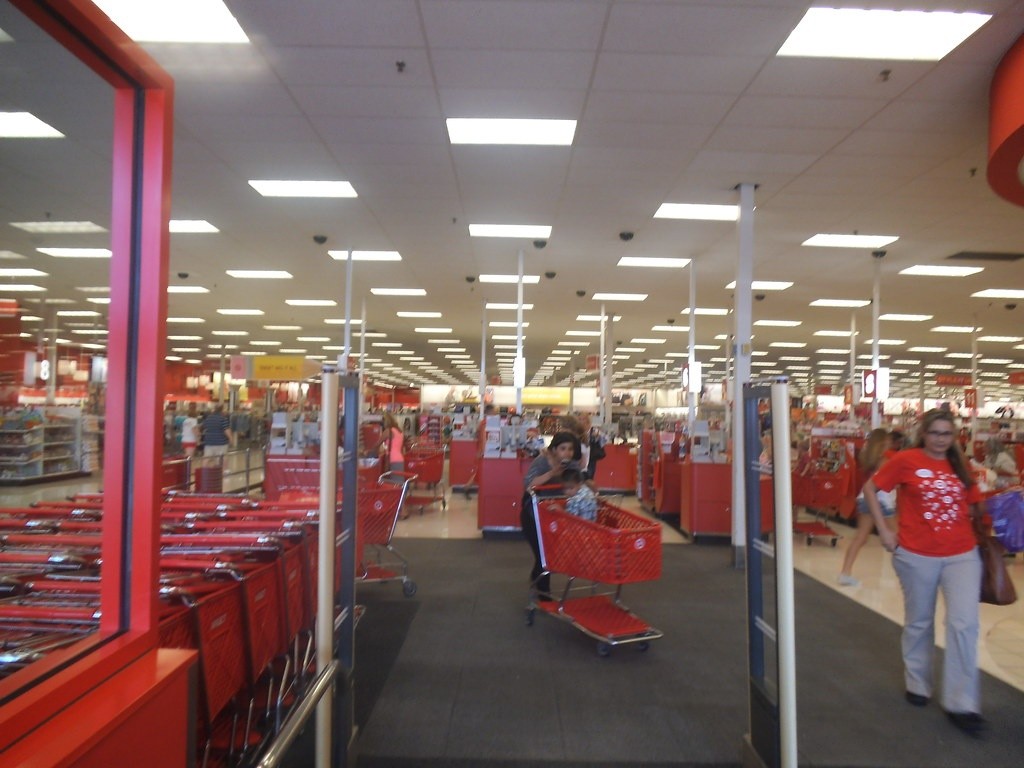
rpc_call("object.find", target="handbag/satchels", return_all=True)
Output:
[589,427,605,461]
[977,538,1017,606]
[382,428,392,479]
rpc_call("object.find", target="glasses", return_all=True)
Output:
[926,431,954,438]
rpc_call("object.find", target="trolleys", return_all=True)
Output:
[403,441,447,515]
[791,468,844,547]
[524,483,664,658]
[0,470,419,768]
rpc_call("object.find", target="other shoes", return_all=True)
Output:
[905,690,930,705]
[837,574,858,586]
[943,709,989,732]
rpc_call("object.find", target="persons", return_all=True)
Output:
[862,408,994,728]
[549,469,597,539]
[181,405,233,474]
[559,412,608,479]
[982,438,1020,557]
[365,413,410,520]
[463,406,496,500]
[520,432,582,606]
[445,386,478,411]
[837,428,905,585]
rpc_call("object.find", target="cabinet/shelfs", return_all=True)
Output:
[0,404,101,486]
